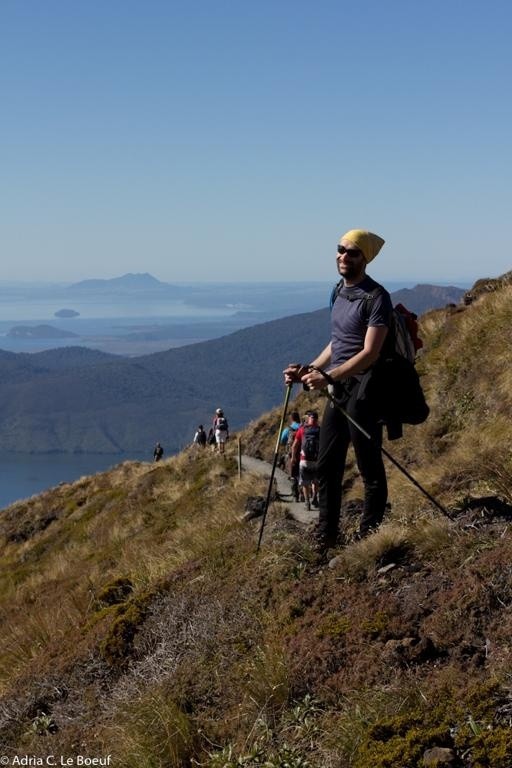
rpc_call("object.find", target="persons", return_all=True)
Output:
[193,408,229,452]
[283,228,398,554]
[280,412,304,502]
[290,411,321,511]
[153,442,163,462]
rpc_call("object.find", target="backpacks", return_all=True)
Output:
[301,425,320,462]
[286,425,296,455]
[214,418,229,431]
[367,296,430,443]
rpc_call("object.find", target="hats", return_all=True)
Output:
[341,228,387,265]
[214,408,223,416]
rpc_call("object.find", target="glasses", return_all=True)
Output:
[335,245,361,259]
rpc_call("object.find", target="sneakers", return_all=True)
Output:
[290,491,318,514]
